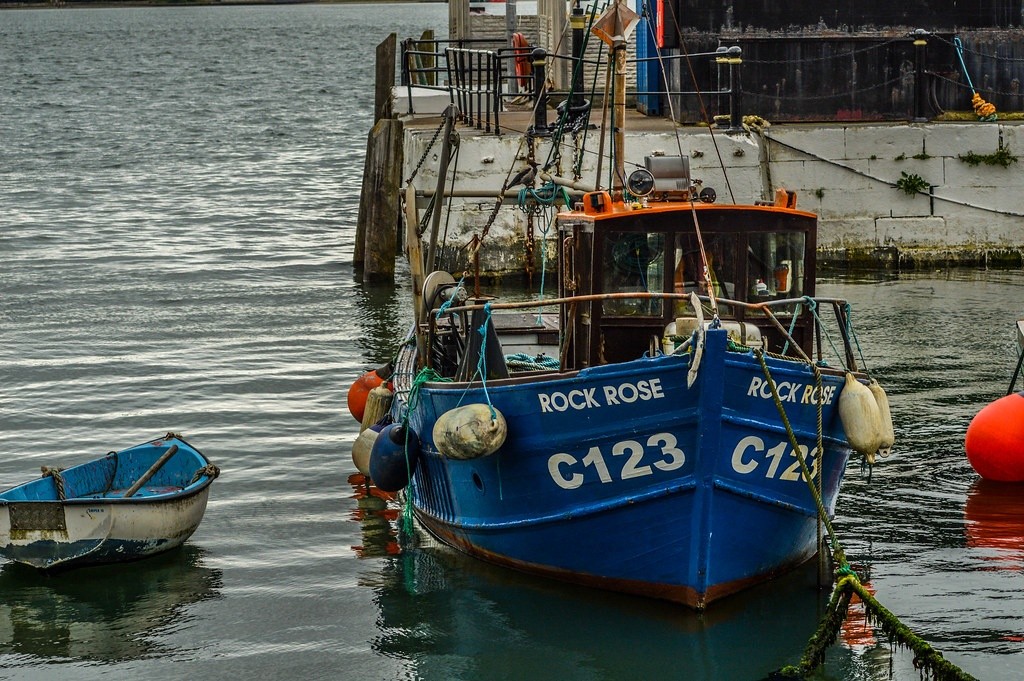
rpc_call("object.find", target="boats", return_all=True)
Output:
[355,2,897,616]
[0,433,218,570]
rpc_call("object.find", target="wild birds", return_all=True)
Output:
[505,160,542,190]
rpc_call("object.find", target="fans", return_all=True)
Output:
[612,232,663,273]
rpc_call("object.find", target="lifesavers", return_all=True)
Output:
[514,28,533,87]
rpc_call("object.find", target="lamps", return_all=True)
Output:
[625,170,655,208]
